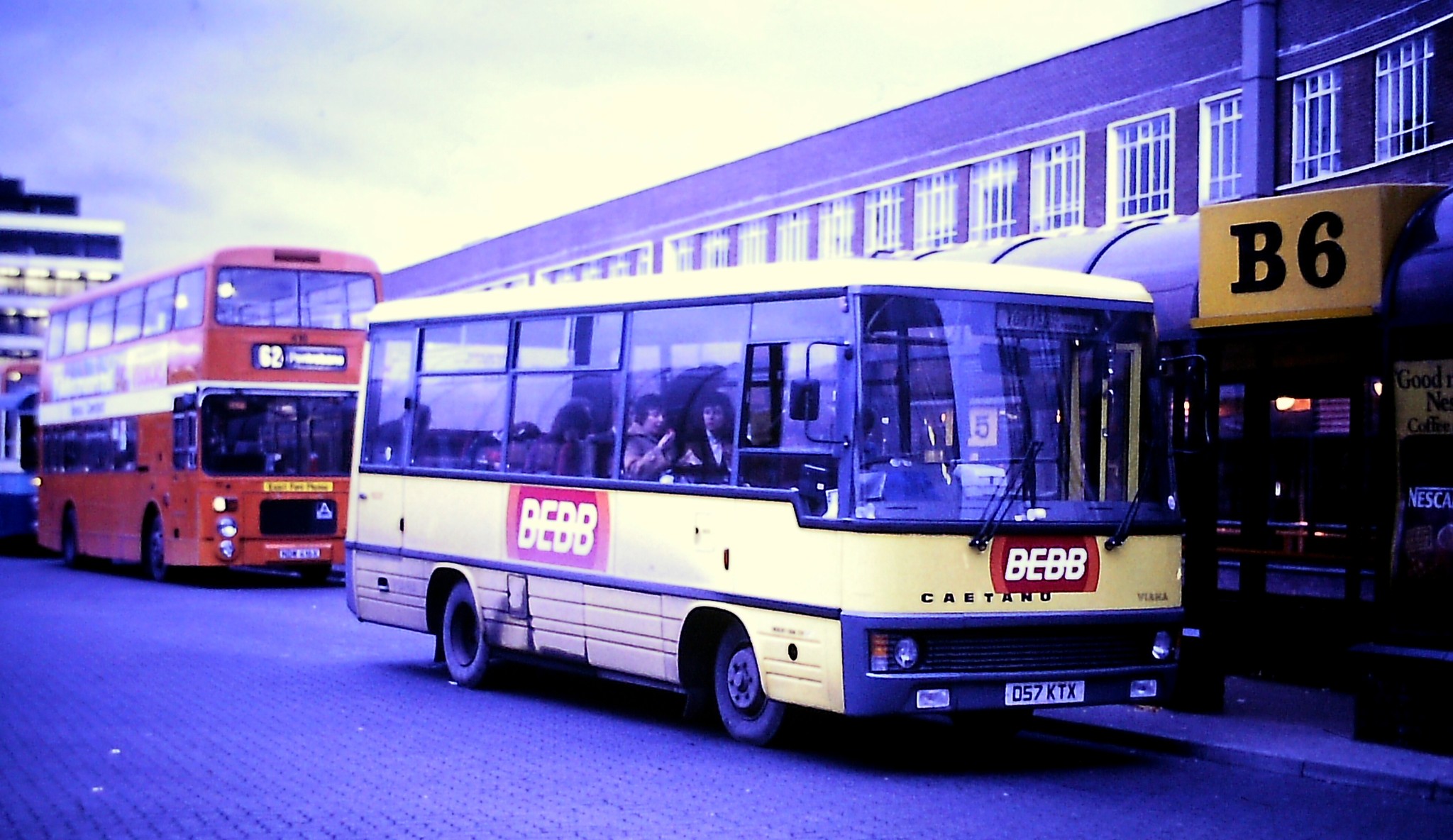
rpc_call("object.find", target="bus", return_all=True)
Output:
[344,257,1181,746]
[37,248,382,580]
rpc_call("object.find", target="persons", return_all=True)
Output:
[200,397,592,480]
[607,390,681,484]
[860,404,881,466]
[586,397,639,478]
[672,391,754,488]
